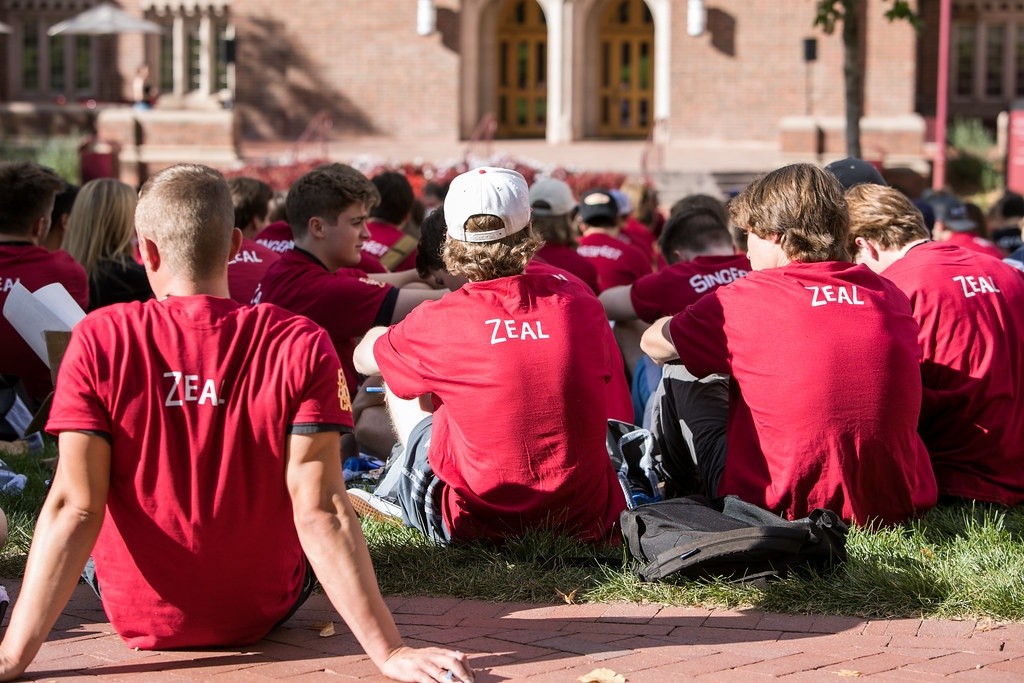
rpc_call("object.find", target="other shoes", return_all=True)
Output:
[345,488,406,526]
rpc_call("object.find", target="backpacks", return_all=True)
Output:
[618,496,850,584]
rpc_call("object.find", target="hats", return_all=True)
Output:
[443,166,530,243]
[581,190,617,222]
[526,177,580,215]
[610,190,633,213]
[824,157,888,193]
[925,191,975,230]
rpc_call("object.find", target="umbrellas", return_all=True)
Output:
[47,1,165,100]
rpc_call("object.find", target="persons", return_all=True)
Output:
[226,158,1024,555]
[133,62,159,146]
[76,108,121,180]
[0,160,153,449]
[0,163,480,683]
